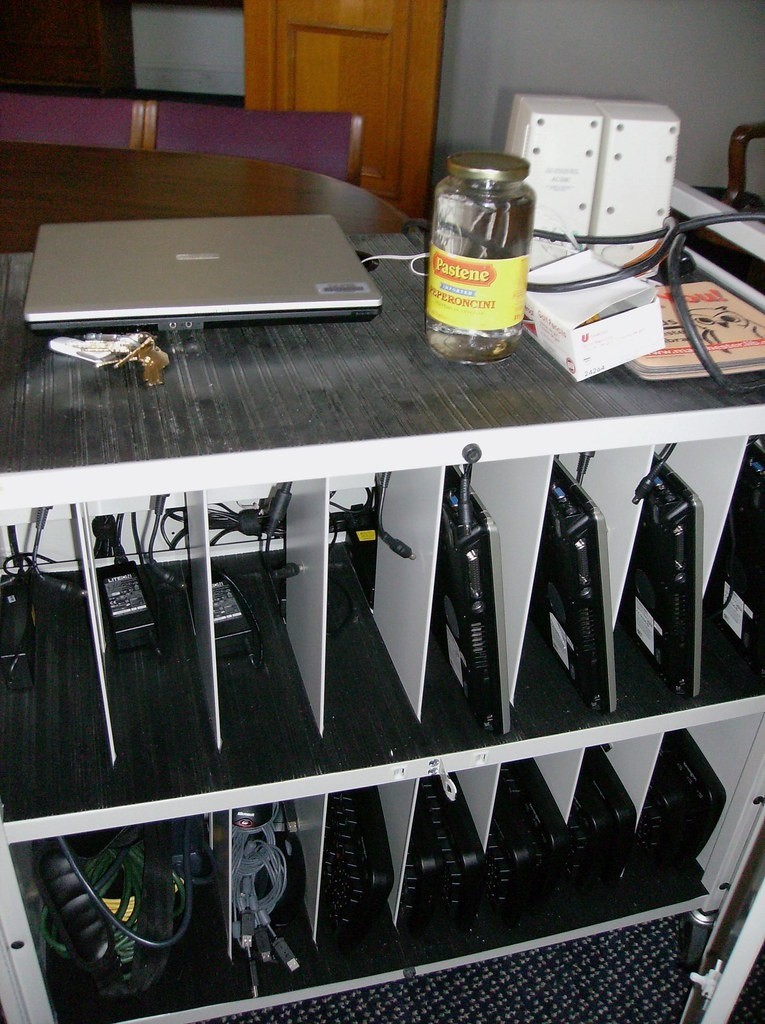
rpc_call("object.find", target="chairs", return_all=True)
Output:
[1,92,366,188]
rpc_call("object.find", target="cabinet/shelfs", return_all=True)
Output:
[0,231,765,1024]
[242,0,447,217]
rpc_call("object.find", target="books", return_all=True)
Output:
[625,281,765,380]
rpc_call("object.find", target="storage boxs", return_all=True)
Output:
[524,248,667,382]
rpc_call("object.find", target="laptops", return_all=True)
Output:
[24,214,381,334]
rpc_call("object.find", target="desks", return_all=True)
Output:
[1,141,419,254]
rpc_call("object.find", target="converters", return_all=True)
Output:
[344,526,379,568]
[207,568,251,657]
[0,583,36,692]
[100,560,159,653]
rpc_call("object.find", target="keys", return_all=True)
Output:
[72,332,169,387]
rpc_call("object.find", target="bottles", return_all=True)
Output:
[424,149,536,366]
